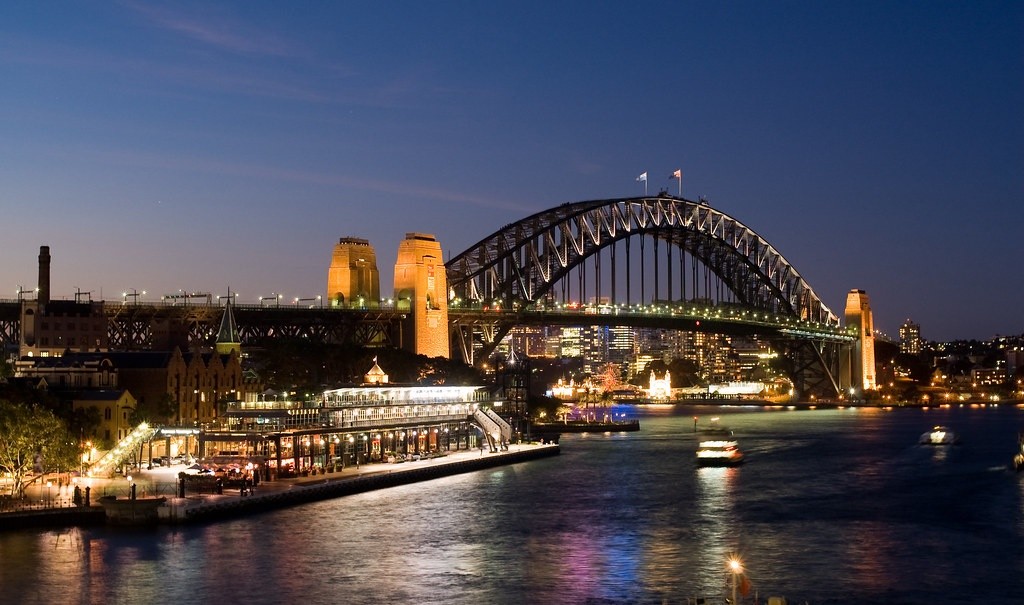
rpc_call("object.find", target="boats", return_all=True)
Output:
[532,387,641,433]
[919,426,956,446]
[696,440,745,465]
